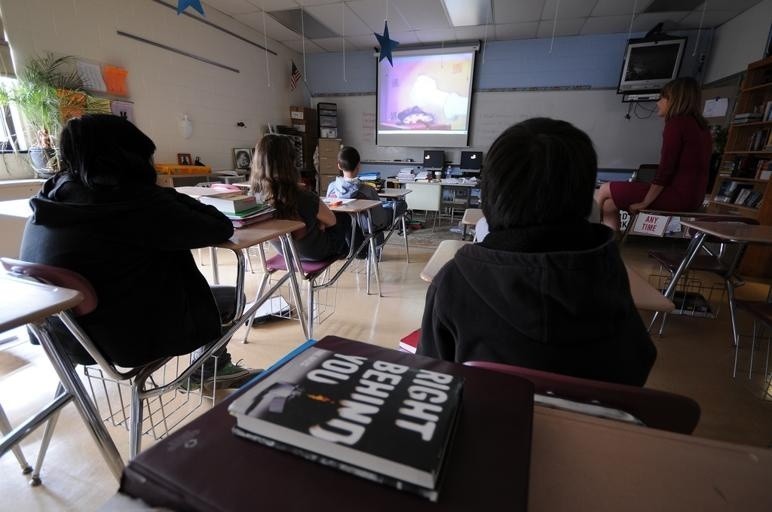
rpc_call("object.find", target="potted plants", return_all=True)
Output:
[0,50,112,178]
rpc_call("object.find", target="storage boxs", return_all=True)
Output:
[290,106,316,132]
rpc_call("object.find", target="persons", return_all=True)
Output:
[16,111,265,395]
[180,155,187,164]
[235,150,249,169]
[417,114,655,386]
[247,133,370,265]
[324,145,407,261]
[589,77,715,244]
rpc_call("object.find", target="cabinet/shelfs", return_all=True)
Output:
[315,102,342,196]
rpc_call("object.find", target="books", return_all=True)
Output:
[228,335,466,501]
[355,171,380,180]
[319,197,358,207]
[170,183,274,229]
[700,94,771,211]
[239,294,291,322]
[404,217,427,230]
[398,326,421,352]
[395,166,431,183]
[658,288,713,318]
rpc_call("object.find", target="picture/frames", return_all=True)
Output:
[233,148,255,169]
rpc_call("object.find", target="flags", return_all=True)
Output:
[287,61,302,93]
[100,62,131,98]
[84,94,114,116]
[110,99,135,124]
[75,60,110,96]
[57,88,87,125]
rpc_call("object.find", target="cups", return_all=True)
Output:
[435,171,442,179]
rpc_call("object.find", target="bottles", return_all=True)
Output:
[445,165,452,180]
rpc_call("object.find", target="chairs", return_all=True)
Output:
[707,58,771,216]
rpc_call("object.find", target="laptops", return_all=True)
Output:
[119,335,536,512]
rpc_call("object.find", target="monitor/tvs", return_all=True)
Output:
[424,150,444,171]
[617,36,688,94]
[459,151,483,173]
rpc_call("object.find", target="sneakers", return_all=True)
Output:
[204,368,265,393]
[178,376,201,394]
